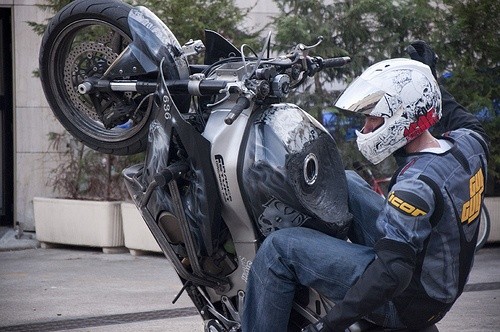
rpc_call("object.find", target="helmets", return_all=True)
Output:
[335,58,444,165]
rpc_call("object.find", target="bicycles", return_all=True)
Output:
[352,157,491,253]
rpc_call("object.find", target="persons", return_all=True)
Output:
[241,40,491,332]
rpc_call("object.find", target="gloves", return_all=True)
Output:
[406,41,440,83]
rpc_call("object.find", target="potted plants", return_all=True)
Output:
[121,144,164,254]
[31,130,123,253]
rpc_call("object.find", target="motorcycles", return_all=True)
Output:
[38,0,440,332]
